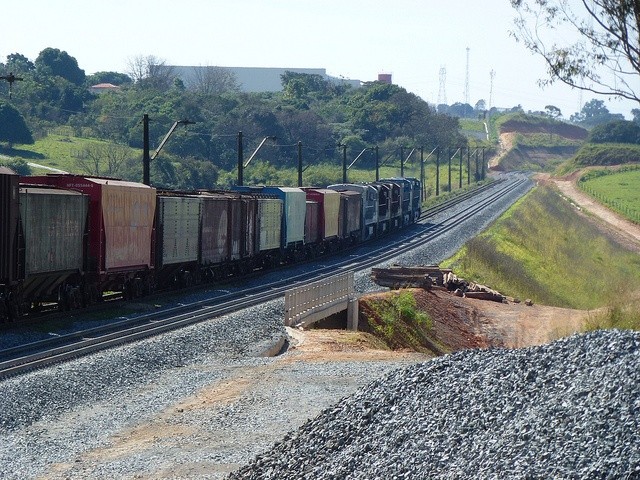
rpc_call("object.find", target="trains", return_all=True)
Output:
[0,174,421,322]
